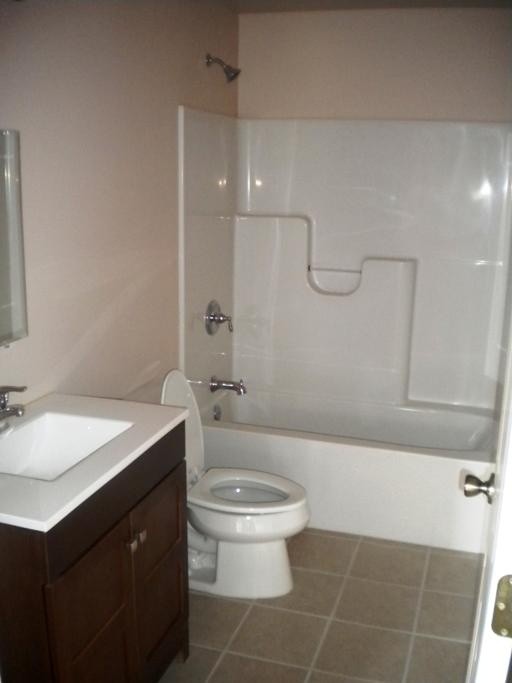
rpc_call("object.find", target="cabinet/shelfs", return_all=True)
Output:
[0,420,190,683]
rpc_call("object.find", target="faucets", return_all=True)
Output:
[0,385,28,434]
[209,375,248,396]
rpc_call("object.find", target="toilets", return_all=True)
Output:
[161,369,308,600]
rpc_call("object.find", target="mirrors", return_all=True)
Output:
[0,127,29,347]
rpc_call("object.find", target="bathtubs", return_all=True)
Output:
[199,386,496,554]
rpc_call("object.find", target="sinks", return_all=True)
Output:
[0,391,190,533]
